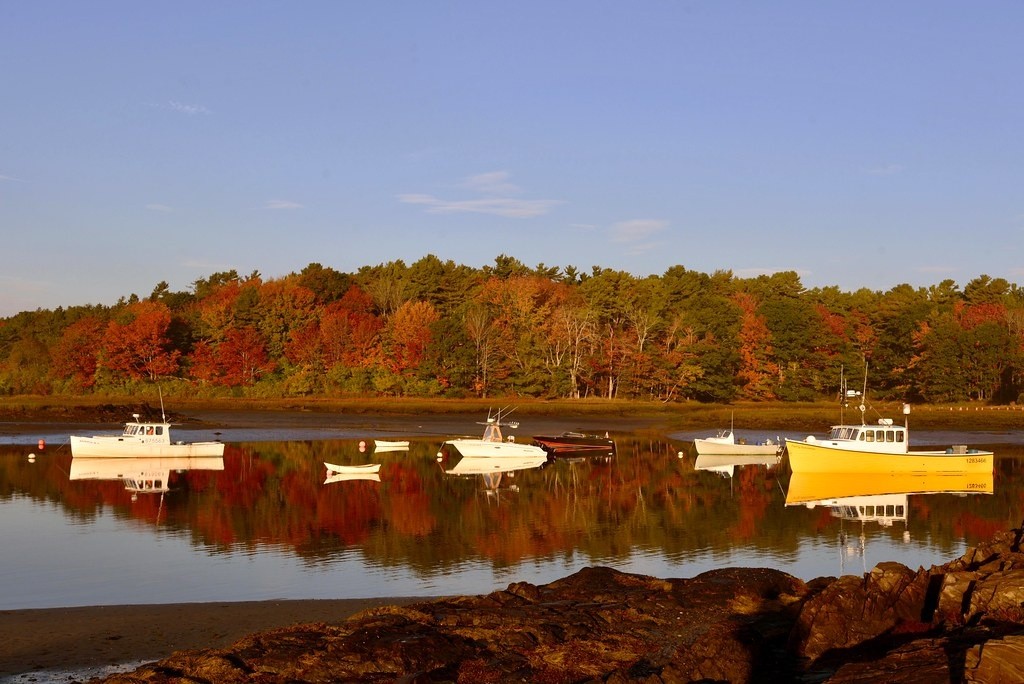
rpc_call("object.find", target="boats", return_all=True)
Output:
[445,404,548,457]
[696,455,782,498]
[374,440,409,446]
[324,461,381,474]
[784,360,995,475]
[68,458,226,528]
[695,413,783,455]
[786,476,995,574]
[71,384,225,459]
[445,457,549,508]
[324,474,382,485]
[374,447,410,453]
